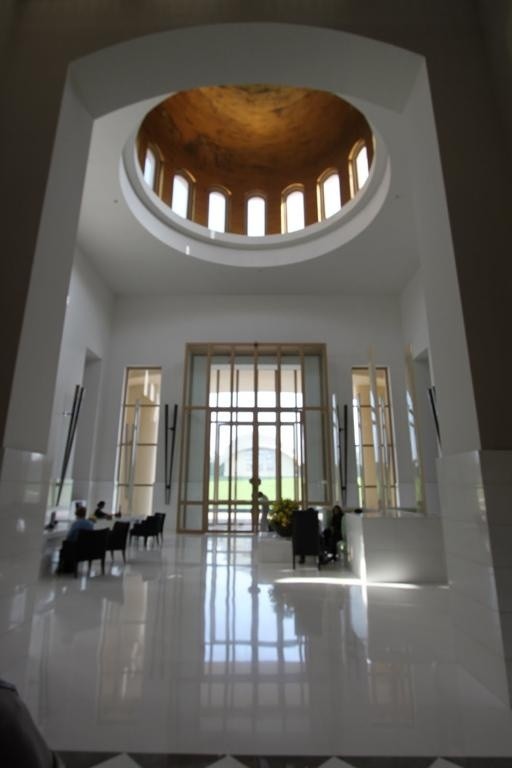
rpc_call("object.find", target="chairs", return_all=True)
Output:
[130,511,166,545]
[292,504,340,569]
[62,518,128,580]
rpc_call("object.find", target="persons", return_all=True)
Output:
[93,500,114,521]
[257,491,272,532]
[64,504,95,544]
[321,504,344,560]
[297,507,329,565]
